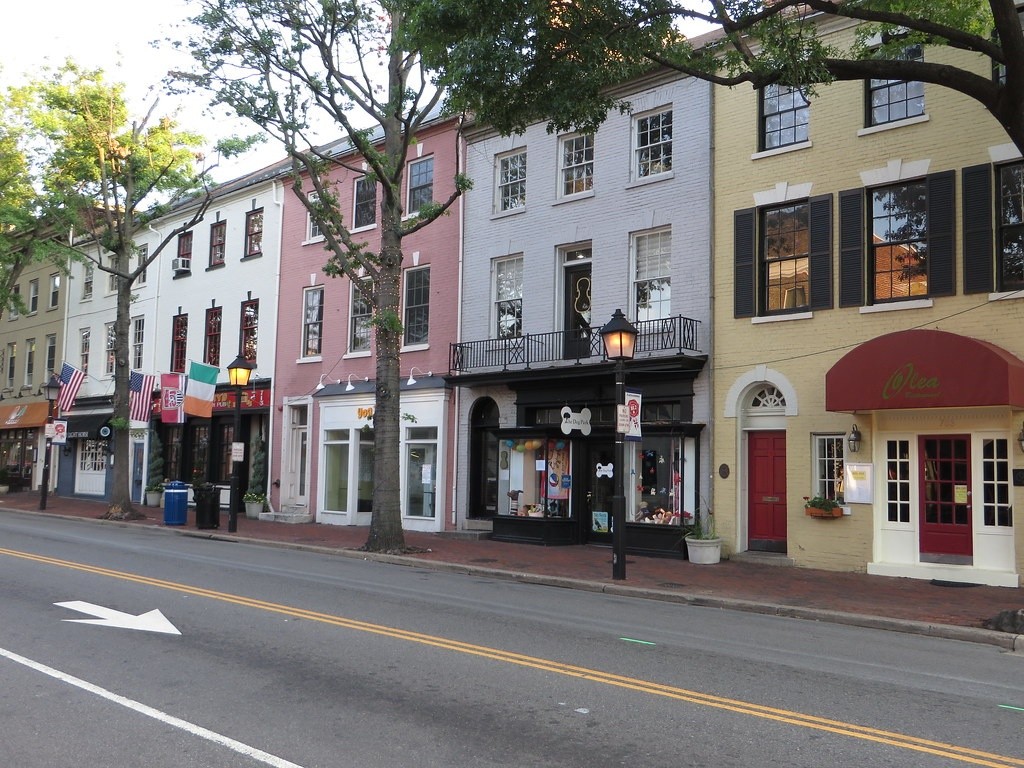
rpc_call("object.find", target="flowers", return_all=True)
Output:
[242,490,266,503]
[802,495,839,512]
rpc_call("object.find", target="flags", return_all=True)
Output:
[129,371,155,422]
[56,363,84,411]
[183,362,219,418]
[160,375,188,424]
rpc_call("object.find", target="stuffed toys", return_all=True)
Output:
[635,501,673,525]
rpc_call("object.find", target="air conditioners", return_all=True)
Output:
[172,258,191,271]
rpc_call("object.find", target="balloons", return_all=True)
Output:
[555,441,565,449]
[512,439,555,452]
[505,440,515,448]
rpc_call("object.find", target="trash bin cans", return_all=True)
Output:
[193,482,223,530]
[163,480,189,526]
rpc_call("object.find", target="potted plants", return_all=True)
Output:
[680,498,722,563]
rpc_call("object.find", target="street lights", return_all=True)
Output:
[39,376,60,511]
[599,309,641,580]
[228,353,253,531]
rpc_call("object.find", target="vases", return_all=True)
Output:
[805,506,843,518]
[244,500,264,519]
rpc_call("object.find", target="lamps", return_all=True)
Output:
[847,423,861,452]
[316,373,340,389]
[407,366,432,385]
[1018,420,1024,453]
[346,373,369,391]
[0,382,47,401]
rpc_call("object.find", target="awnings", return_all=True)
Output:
[0,402,49,429]
[60,415,113,440]
